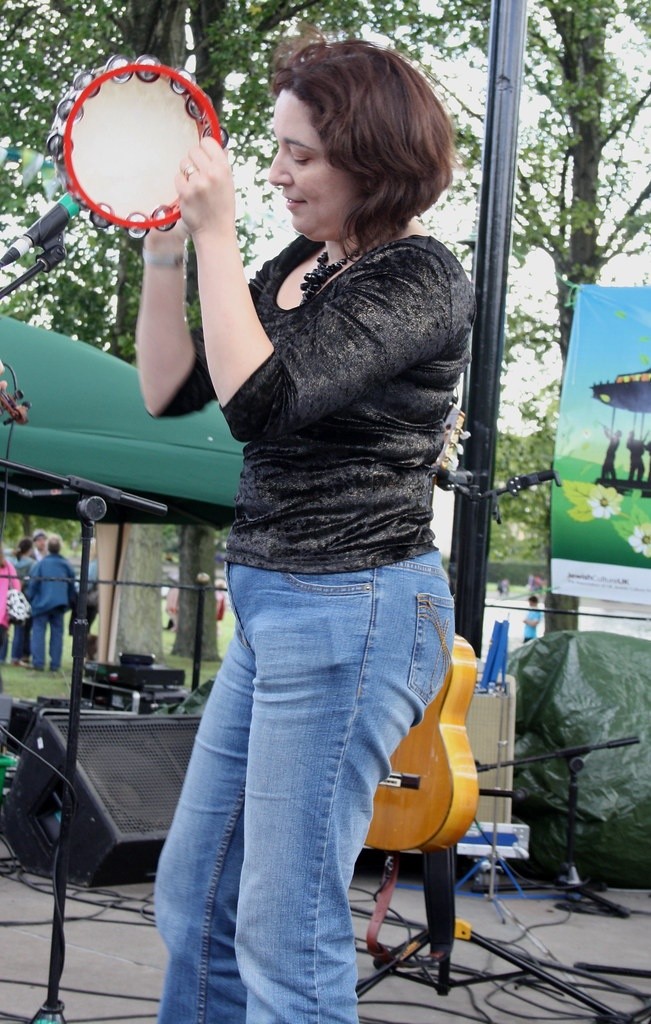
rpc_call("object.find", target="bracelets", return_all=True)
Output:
[142,246,188,267]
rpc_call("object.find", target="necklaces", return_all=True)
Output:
[300,240,365,306]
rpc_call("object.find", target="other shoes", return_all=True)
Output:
[22,656,29,662]
[12,660,34,669]
[31,666,44,671]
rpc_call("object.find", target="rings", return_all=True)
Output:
[182,164,198,180]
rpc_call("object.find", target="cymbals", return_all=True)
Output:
[46,54,229,241]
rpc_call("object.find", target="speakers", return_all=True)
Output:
[0,707,204,884]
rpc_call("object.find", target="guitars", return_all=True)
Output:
[362,629,479,853]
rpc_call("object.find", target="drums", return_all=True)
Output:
[58,63,225,227]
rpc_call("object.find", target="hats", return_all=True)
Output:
[32,529,48,541]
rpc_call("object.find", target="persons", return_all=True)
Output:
[522,597,542,644]
[0,359,8,392]
[0,529,99,672]
[134,34,478,1024]
[528,573,545,592]
[498,577,512,598]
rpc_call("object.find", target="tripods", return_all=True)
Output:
[356,734,651,1024]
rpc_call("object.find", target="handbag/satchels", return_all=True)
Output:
[6,589,32,625]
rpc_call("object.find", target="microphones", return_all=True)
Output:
[0,193,89,268]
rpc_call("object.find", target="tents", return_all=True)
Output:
[0,315,248,528]
